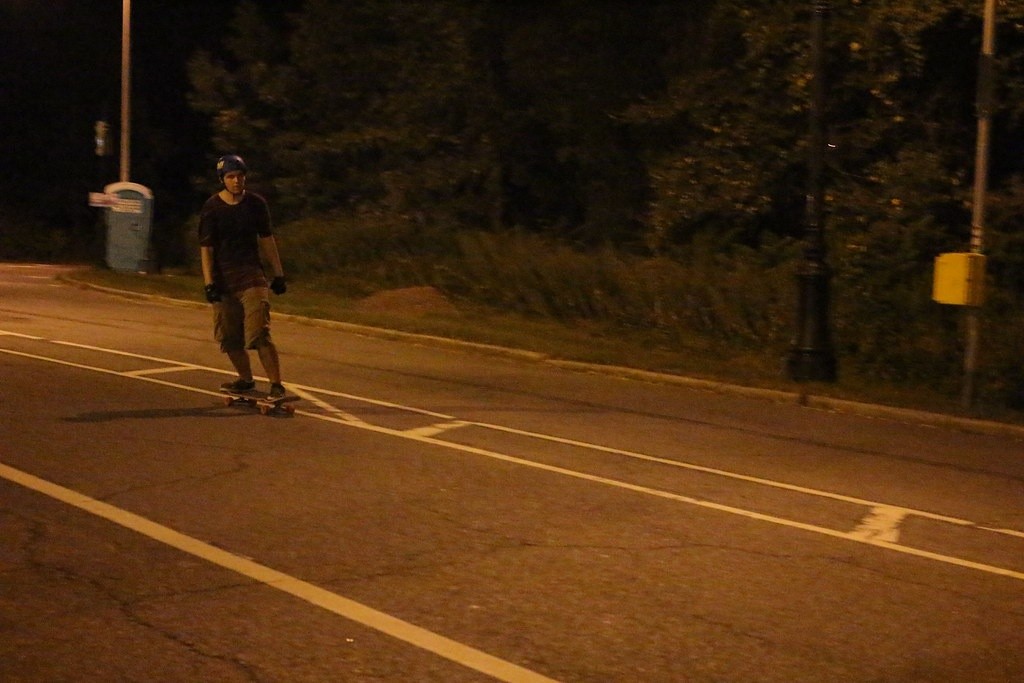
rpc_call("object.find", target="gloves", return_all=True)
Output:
[269,276,287,296]
[205,282,224,304]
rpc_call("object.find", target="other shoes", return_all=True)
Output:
[267,383,287,400]
[219,378,256,394]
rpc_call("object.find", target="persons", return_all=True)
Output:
[82,117,120,237]
[199,156,287,400]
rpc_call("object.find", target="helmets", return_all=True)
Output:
[216,155,247,177]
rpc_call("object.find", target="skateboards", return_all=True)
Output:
[218,382,301,415]
